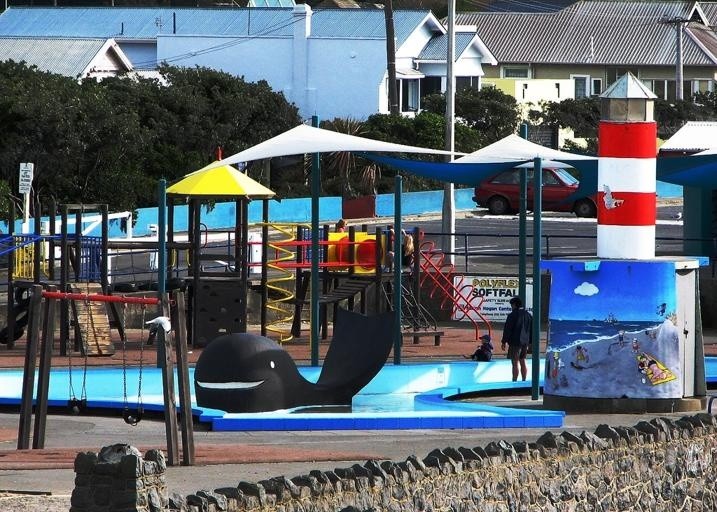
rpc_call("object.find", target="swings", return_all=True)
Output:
[66,298,91,416]
[120,302,148,425]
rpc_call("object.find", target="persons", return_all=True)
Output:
[501,294,533,382]
[546,300,673,389]
[461,334,495,363]
[333,218,415,272]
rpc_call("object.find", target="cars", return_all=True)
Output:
[471,166,597,218]
